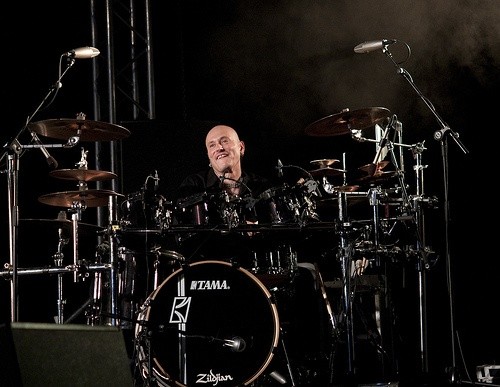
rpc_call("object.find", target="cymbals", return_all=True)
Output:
[308,168,348,177]
[310,159,340,166]
[18,218,106,232]
[358,171,407,184]
[48,168,118,182]
[304,107,391,137]
[359,161,390,172]
[28,117,132,143]
[38,188,130,208]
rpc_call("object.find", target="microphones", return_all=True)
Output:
[355,38,398,53]
[65,46,100,58]
[373,117,392,165]
[154,173,158,188]
[27,128,58,169]
[277,159,284,177]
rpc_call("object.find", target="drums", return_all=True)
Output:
[120,196,168,251]
[103,249,187,332]
[135,261,280,387]
[243,246,298,276]
[249,185,321,232]
[160,196,245,259]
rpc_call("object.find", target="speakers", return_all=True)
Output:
[0,322,132,387]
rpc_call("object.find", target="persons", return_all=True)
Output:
[173,123,273,244]
